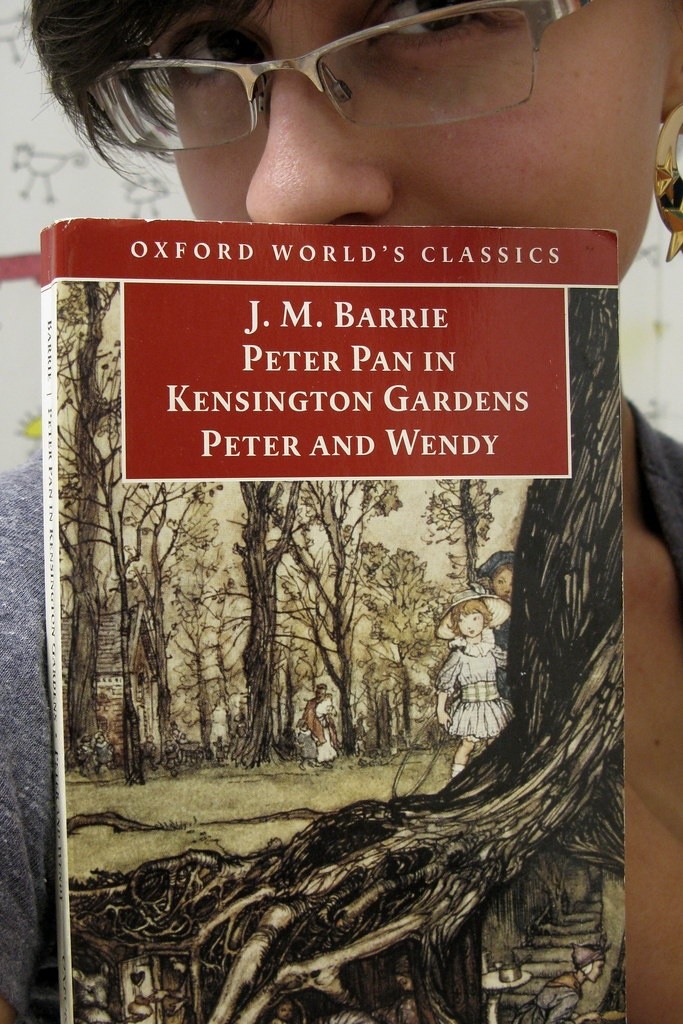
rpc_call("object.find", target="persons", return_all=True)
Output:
[32,1,683,1024]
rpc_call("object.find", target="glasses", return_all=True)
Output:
[87,0,592,152]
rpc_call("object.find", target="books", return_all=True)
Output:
[0,219,631,1024]
